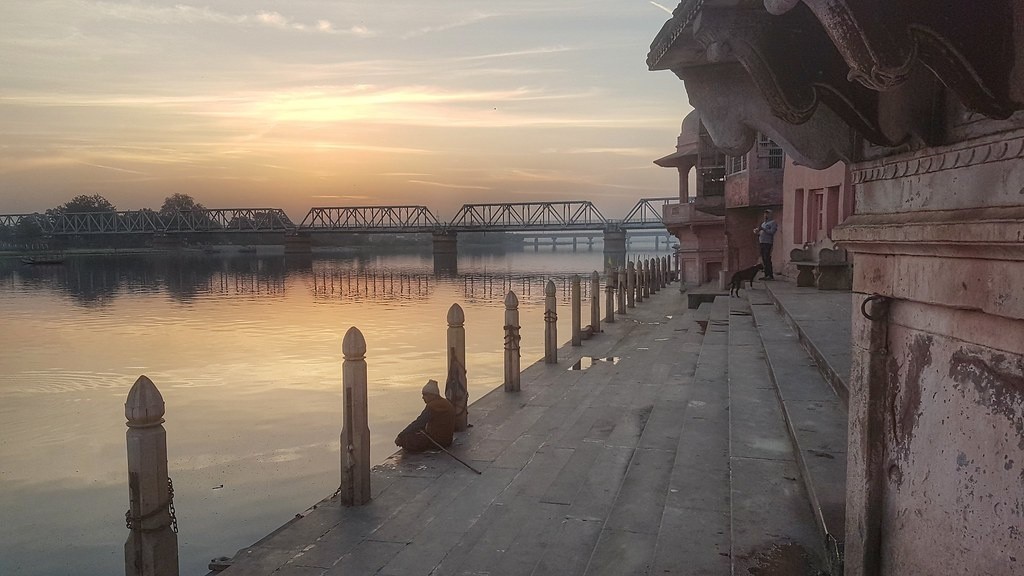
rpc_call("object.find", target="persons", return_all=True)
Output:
[754,210,777,281]
[395,380,455,453]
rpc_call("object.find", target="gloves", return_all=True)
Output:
[395,436,404,447]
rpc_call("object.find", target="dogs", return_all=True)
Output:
[727,262,764,298]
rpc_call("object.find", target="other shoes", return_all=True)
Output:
[758,275,775,281]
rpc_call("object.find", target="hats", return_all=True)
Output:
[422,379,440,396]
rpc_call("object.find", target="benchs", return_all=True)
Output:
[789,237,851,290]
